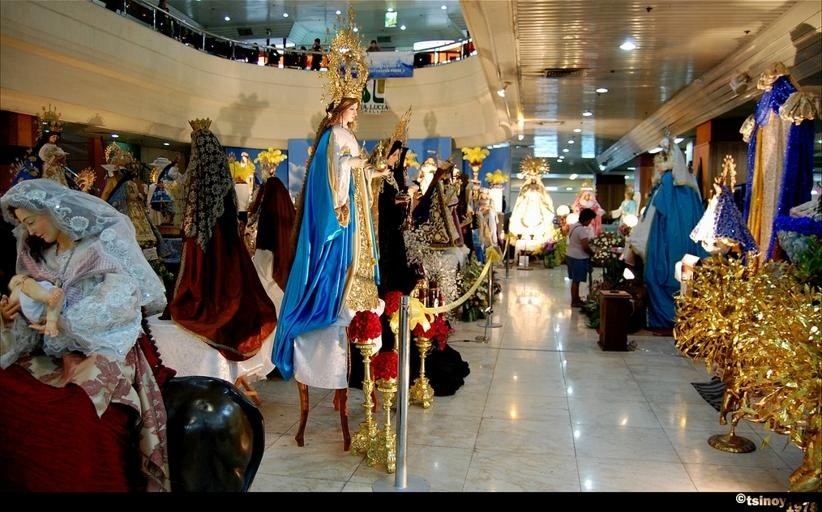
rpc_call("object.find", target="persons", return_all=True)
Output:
[0,177,174,495]
[93,1,330,78]
[0,64,822,398]
[366,40,380,52]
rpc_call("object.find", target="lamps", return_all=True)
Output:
[602,158,611,166]
[497,82,511,97]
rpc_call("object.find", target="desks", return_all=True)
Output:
[599,289,635,350]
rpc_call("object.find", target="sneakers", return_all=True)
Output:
[572,300,585,307]
